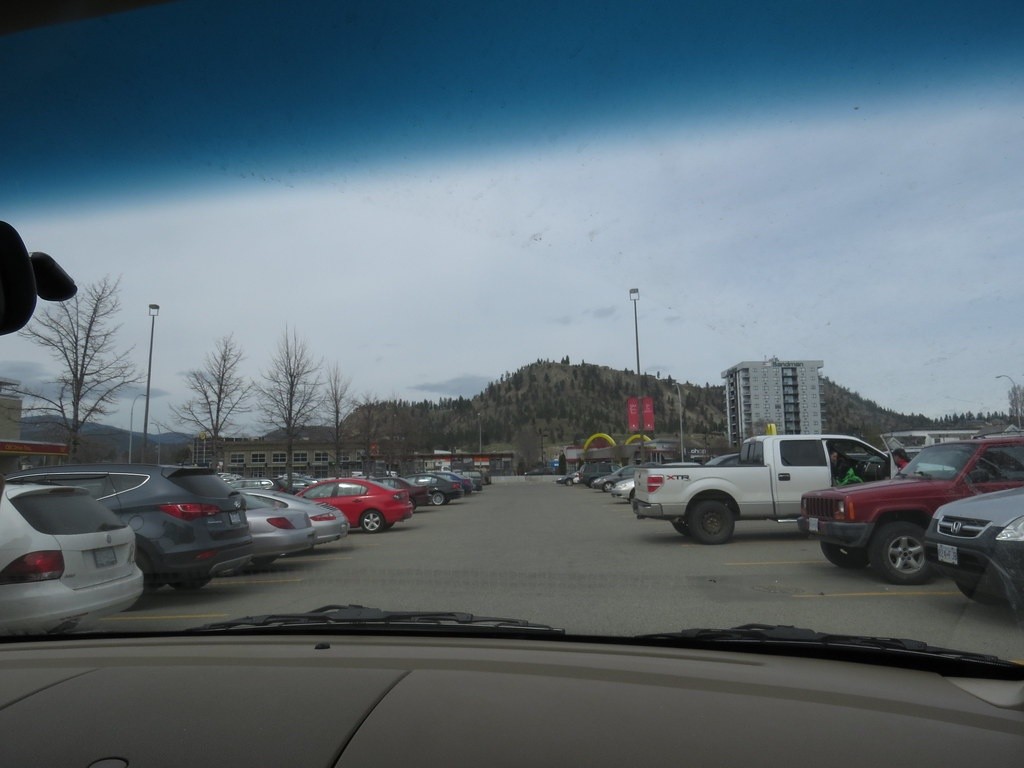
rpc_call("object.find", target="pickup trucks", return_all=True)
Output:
[627,432,956,545]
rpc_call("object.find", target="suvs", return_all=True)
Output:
[798,430,1024,587]
[0,462,254,613]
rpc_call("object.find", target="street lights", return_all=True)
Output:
[629,287,646,465]
[138,303,160,465]
[995,374,1022,430]
[671,383,685,462]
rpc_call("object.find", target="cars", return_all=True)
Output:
[275,476,414,534]
[556,452,802,504]
[219,466,492,515]
[371,476,435,512]
[919,485,1024,610]
[0,479,146,637]
[235,488,350,557]
[238,491,318,573]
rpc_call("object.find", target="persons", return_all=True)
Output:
[893,448,915,476]
[829,448,846,486]
